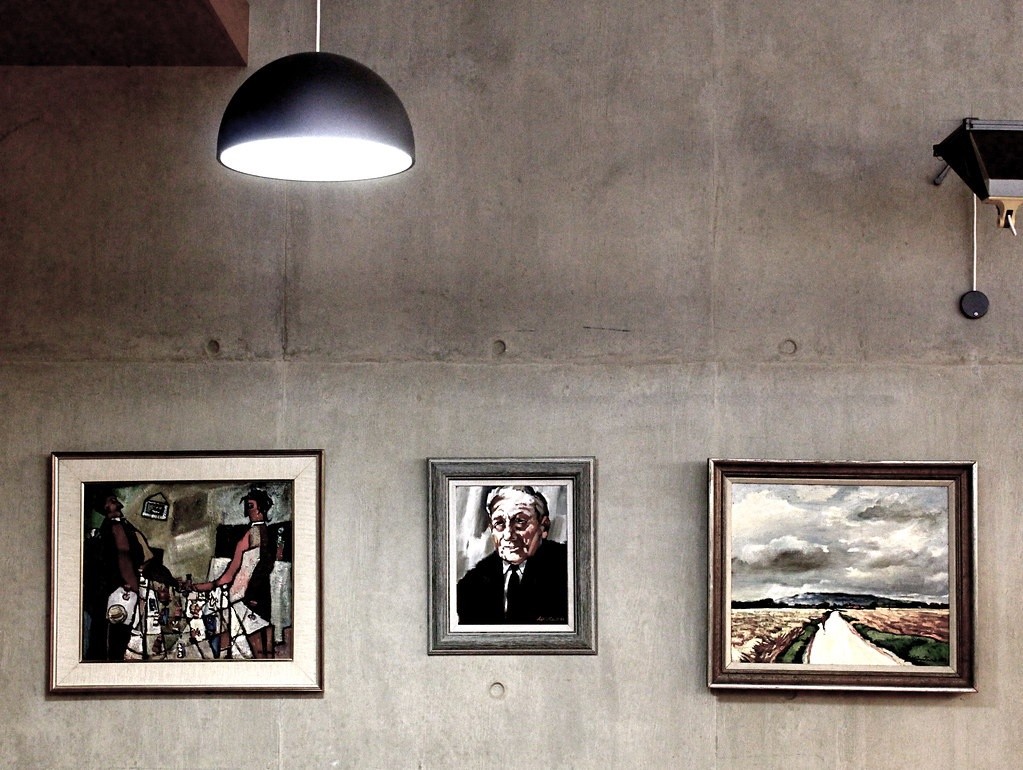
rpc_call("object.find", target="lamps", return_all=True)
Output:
[216,1,415,182]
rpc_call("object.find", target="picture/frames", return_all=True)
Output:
[707,456,984,696]
[49,446,326,696]
[427,458,598,655]
